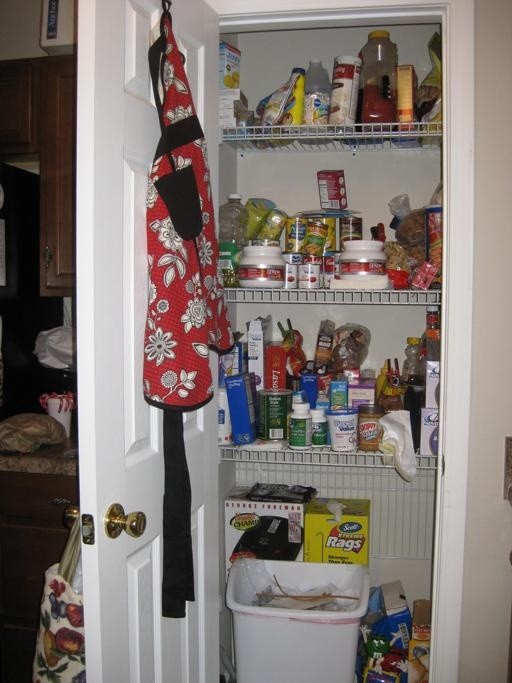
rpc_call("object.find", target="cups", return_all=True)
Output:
[46,394,75,439]
[326,410,358,451]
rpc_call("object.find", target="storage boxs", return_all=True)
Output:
[224,485,304,583]
[304,497,371,566]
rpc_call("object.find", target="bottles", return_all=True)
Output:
[421,306,442,387]
[328,53,363,130]
[402,337,424,384]
[338,240,388,289]
[359,359,403,452]
[289,403,312,450]
[310,408,327,444]
[239,239,286,287]
[219,192,250,288]
[302,59,331,128]
[358,29,399,131]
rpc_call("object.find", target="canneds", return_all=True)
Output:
[282,250,340,292]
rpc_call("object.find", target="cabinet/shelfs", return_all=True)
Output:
[217,121,443,470]
[0,471,80,681]
[0,54,77,298]
